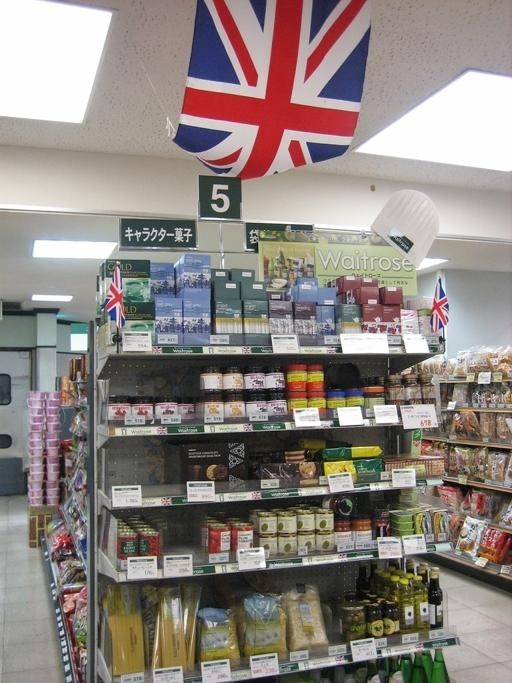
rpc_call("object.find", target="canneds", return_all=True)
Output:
[106,363,435,423]
[341,590,400,642]
[115,504,371,564]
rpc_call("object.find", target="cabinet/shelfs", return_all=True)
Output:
[40,322,512,683]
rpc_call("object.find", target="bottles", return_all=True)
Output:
[356,557,444,639]
[306,649,451,683]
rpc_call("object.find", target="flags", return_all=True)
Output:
[165,0,372,179]
[100,265,127,330]
[429,271,451,332]
